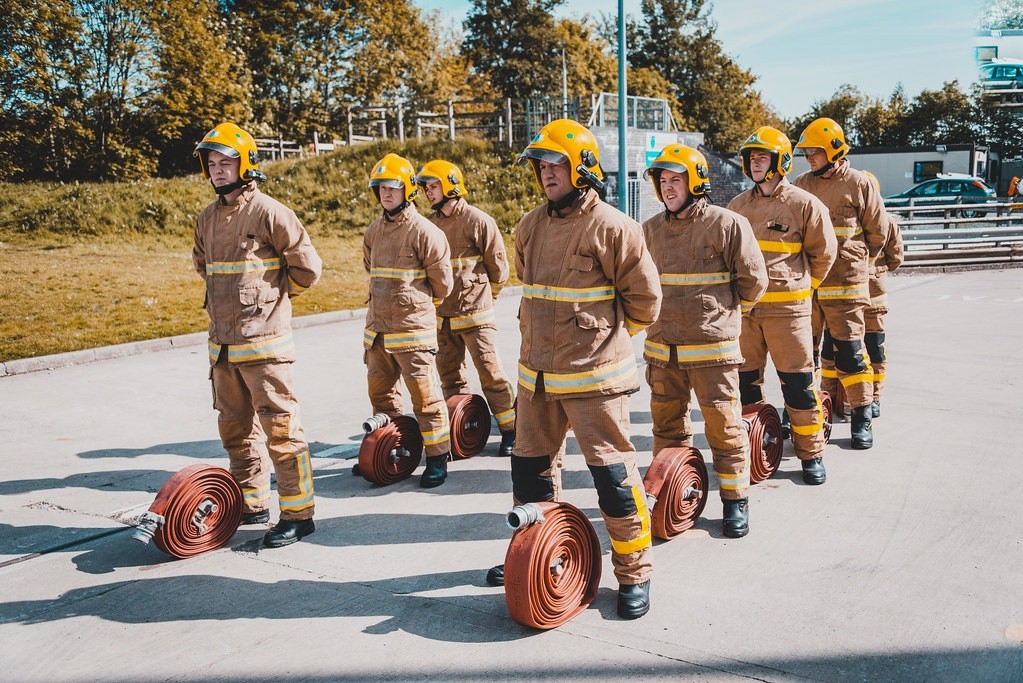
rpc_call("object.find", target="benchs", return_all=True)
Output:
[888,201,1023,265]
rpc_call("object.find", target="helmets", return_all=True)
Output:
[643,144,712,200]
[739,126,793,176]
[793,118,849,163]
[518,119,603,188]
[368,154,419,202]
[193,123,268,182]
[861,170,880,195]
[418,159,468,198]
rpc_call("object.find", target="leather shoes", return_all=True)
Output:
[872,402,880,416]
[802,459,825,483]
[486,566,505,585]
[351,464,360,475]
[240,509,269,523]
[851,407,873,446]
[722,500,749,537]
[780,410,790,436]
[420,457,446,488]
[263,519,315,547]
[617,580,649,619]
[500,433,514,453]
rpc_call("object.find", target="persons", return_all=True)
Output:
[486,118,662,620]
[792,118,889,449]
[417,159,515,456]
[642,144,769,538]
[351,157,450,489]
[726,127,838,484]
[820,169,904,419]
[191,121,322,546]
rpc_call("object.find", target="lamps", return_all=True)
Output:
[935,144,947,153]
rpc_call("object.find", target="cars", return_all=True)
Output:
[976,63,1023,103]
[881,177,999,219]
